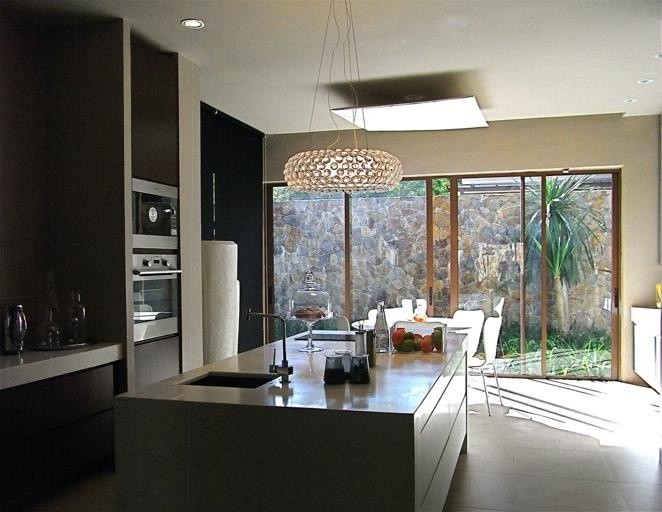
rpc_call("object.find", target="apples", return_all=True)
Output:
[392,330,442,353]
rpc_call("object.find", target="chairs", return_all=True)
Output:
[367,297,504,417]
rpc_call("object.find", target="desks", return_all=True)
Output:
[351,317,472,331]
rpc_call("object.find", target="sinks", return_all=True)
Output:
[175,371,282,389]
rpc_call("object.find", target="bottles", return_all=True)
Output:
[375,299,390,352]
[36,271,88,347]
[324,349,369,385]
[355,331,364,352]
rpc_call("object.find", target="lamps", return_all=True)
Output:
[330,95,489,133]
[282,0,403,195]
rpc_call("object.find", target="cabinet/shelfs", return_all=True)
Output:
[1,362,115,509]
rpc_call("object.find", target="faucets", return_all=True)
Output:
[244,308,287,370]
[271,375,290,408]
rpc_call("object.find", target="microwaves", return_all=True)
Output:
[127,179,180,253]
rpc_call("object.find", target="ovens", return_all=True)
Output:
[127,255,182,342]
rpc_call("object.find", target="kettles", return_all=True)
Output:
[0,302,27,355]
